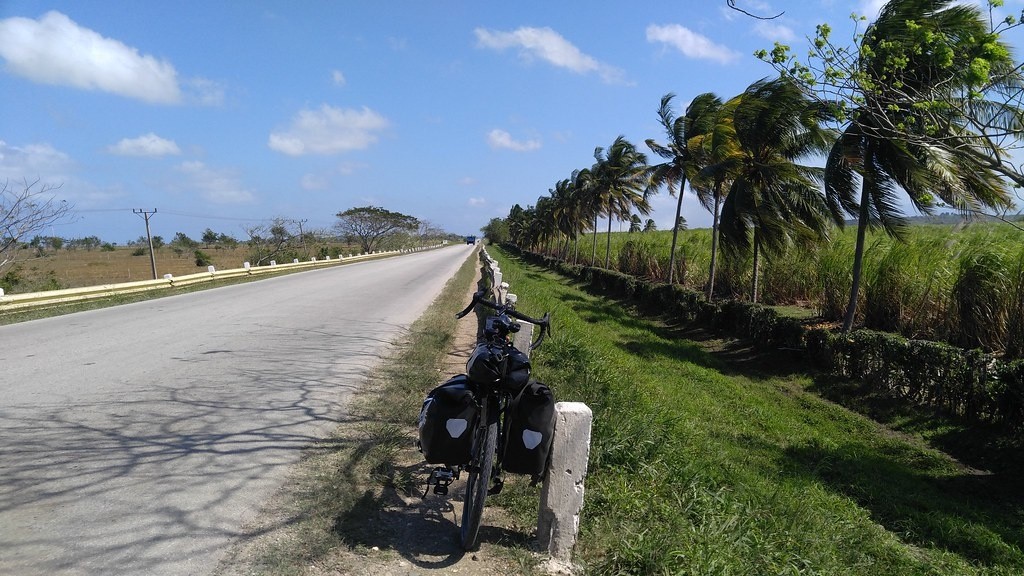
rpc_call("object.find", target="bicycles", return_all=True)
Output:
[430,286,553,552]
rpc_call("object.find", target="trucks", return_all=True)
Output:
[466,236,475,245]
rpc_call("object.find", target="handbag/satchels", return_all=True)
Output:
[467,342,532,398]
[500,382,557,476]
[419,377,482,466]
